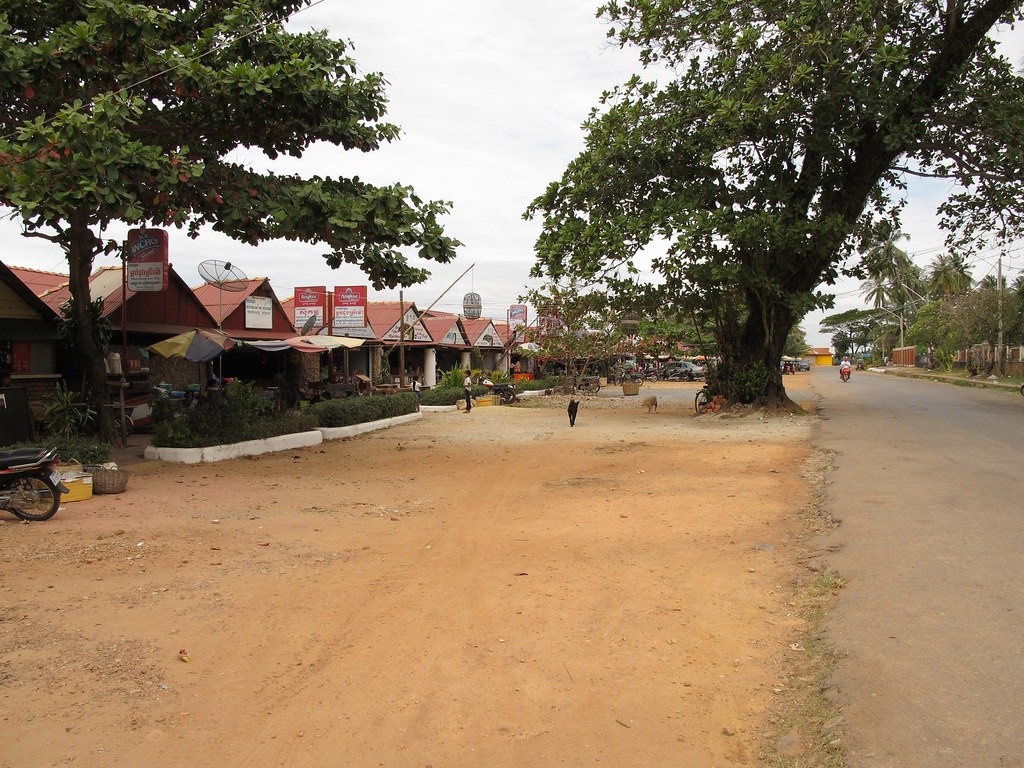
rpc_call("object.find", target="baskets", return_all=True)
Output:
[85,466,130,493]
[55,458,84,475]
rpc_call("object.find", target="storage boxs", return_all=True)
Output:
[27,459,93,504]
[475,394,501,407]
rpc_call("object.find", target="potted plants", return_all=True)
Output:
[623,363,639,396]
[598,361,608,387]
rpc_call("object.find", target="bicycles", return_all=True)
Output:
[695,382,708,411]
[77,395,133,437]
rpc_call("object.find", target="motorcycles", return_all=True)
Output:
[477,374,517,405]
[0,447,70,521]
[575,372,602,392]
[617,365,659,386]
[782,358,795,374]
[840,365,851,382]
[857,361,863,371]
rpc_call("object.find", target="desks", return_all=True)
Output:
[376,384,397,395]
[402,384,430,392]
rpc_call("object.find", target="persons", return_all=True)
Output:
[410,375,421,412]
[463,370,471,413]
[840,356,851,379]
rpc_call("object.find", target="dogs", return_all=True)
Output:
[643,396,658,414]
[567,400,580,427]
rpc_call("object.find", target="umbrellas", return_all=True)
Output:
[148,327,242,383]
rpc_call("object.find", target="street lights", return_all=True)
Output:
[957,246,1003,375]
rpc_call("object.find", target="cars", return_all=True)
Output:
[661,362,706,380]
[798,360,810,371]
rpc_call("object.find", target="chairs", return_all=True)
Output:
[583,378,599,396]
